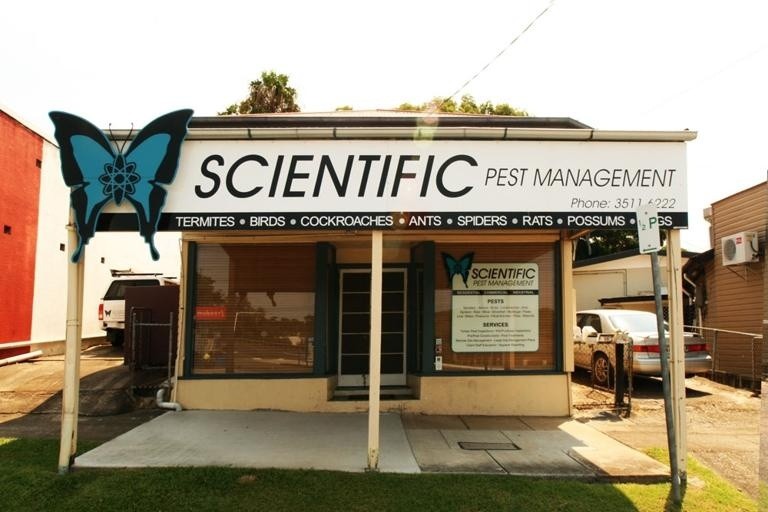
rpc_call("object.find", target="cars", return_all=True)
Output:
[572,308,714,388]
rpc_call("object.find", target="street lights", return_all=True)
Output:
[233,309,275,333]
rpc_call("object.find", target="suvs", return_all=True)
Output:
[96,270,176,351]
